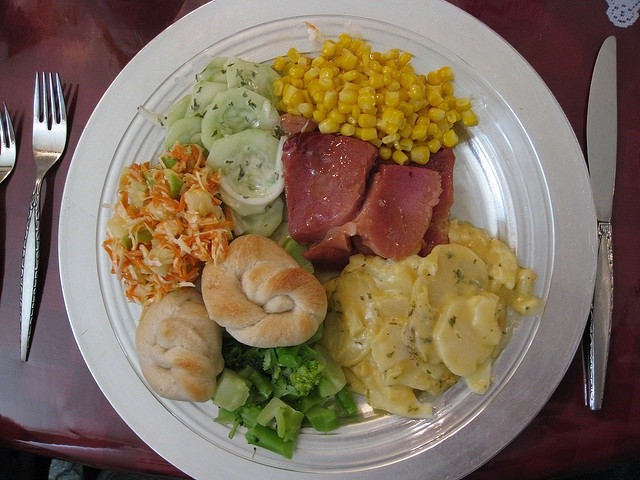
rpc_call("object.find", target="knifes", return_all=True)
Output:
[581,35,618,410]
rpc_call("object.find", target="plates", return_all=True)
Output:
[58,0,598,478]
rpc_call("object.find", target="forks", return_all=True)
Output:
[1,100,17,185]
[19,72,69,363]
